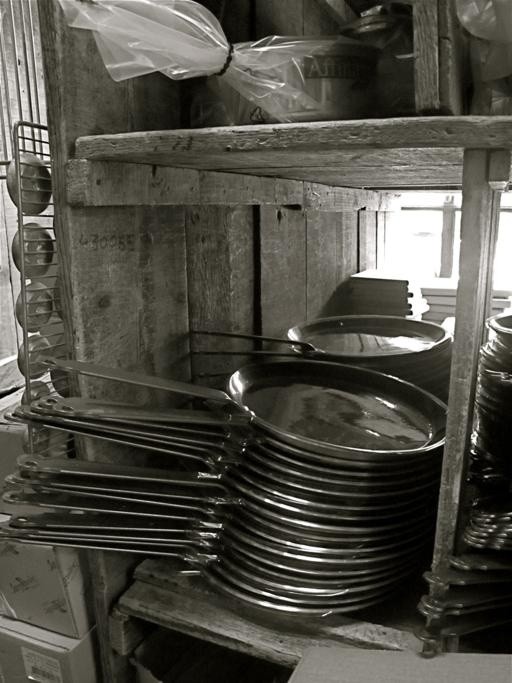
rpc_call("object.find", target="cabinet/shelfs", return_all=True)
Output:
[38,2,510,683]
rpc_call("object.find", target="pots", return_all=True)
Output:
[168,3,383,127]
[179,314,454,400]
[415,308,511,660]
[0,354,447,617]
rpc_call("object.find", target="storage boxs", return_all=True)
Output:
[0,528,95,639]
[0,617,103,682]
